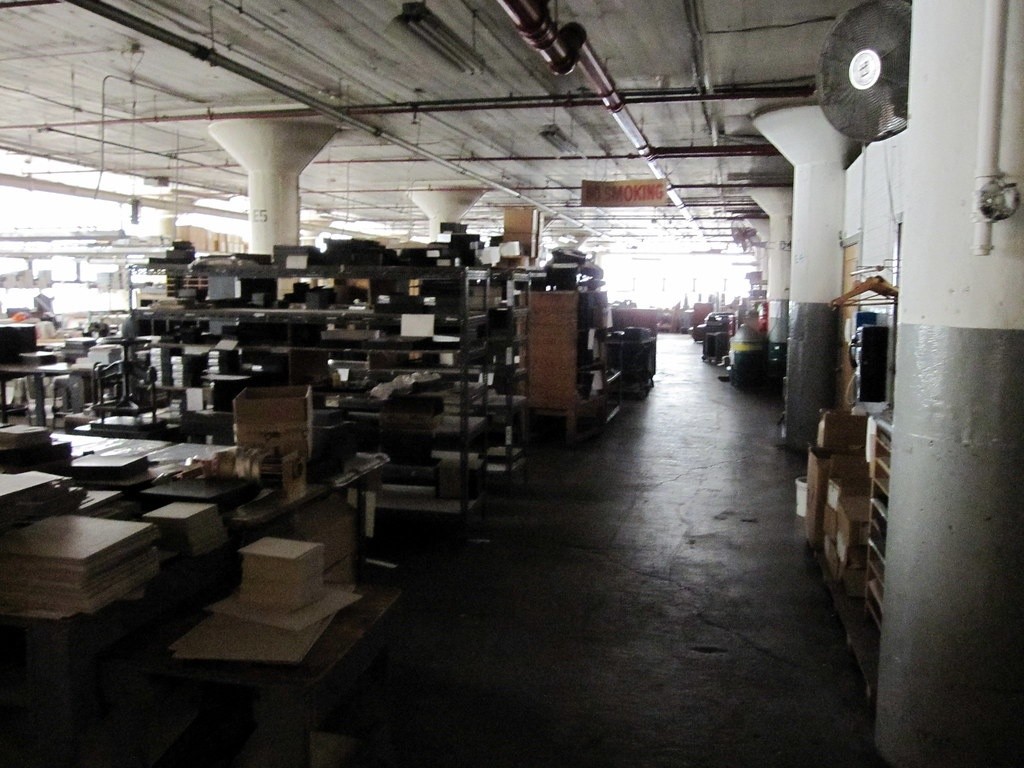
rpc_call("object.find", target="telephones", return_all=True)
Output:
[848,325,887,402]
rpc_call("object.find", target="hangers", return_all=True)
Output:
[828,272,898,312]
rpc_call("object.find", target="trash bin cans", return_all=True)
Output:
[728,339,766,392]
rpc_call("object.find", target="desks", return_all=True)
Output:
[0,337,152,428]
[609,307,662,334]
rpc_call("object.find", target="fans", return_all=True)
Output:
[816,0,912,141]
[729,218,770,251]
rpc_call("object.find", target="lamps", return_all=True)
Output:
[383,0,485,89]
[538,106,587,160]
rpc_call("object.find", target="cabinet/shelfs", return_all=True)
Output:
[95,223,622,561]
[863,409,893,632]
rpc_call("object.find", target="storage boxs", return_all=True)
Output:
[804,409,868,594]
[232,386,314,458]
